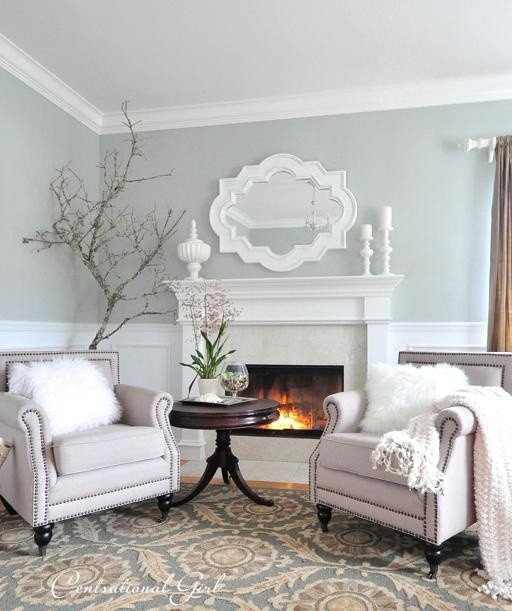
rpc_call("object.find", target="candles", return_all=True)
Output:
[380,206,392,227]
[360,224,373,240]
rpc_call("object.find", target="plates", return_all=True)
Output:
[179,396,257,406]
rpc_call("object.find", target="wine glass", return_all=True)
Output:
[219,360,250,398]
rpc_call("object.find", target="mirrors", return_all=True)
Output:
[209,153,357,274]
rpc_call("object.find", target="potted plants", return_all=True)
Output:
[180,309,237,395]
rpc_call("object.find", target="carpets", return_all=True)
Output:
[2,482,511,609]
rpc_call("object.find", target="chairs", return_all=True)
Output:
[308,351,511,576]
[1,347,180,557]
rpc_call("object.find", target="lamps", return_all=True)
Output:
[305,186,330,233]
